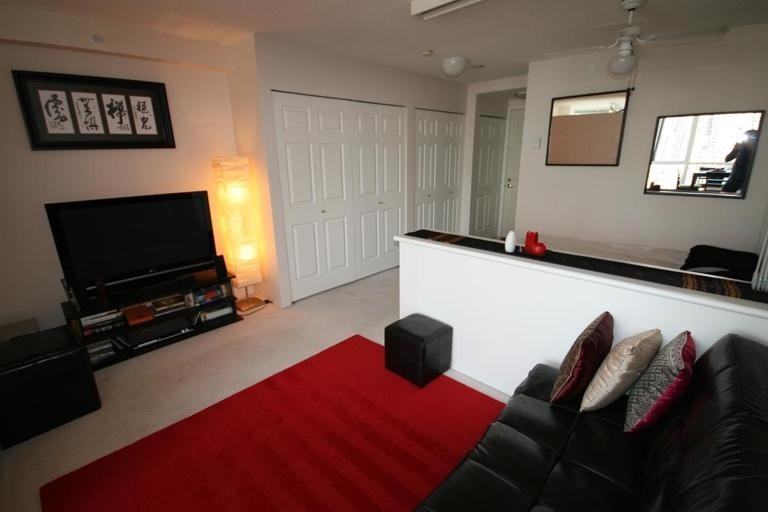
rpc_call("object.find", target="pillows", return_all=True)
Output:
[580,328,661,412]
[550,310,614,403]
[623,331,696,432]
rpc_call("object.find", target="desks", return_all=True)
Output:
[691,172,731,187]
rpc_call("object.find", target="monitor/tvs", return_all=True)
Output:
[43,190,217,298]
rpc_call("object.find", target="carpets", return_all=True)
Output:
[40,333,506,512]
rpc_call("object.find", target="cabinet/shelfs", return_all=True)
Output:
[60,273,243,371]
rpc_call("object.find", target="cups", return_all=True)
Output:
[531,242,545,256]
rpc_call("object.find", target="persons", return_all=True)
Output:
[722,129,758,193]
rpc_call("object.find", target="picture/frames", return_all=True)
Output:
[11,69,177,150]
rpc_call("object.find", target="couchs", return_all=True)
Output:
[411,331,768,512]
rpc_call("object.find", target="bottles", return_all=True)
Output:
[505,230,517,253]
[524,230,538,254]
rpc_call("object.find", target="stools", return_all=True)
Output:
[0,325,103,449]
[384,314,453,388]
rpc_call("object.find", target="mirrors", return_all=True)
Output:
[644,110,764,199]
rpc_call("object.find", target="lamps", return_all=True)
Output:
[608,0,646,75]
[208,156,265,313]
[440,57,468,77]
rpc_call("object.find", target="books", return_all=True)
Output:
[80,284,233,361]
